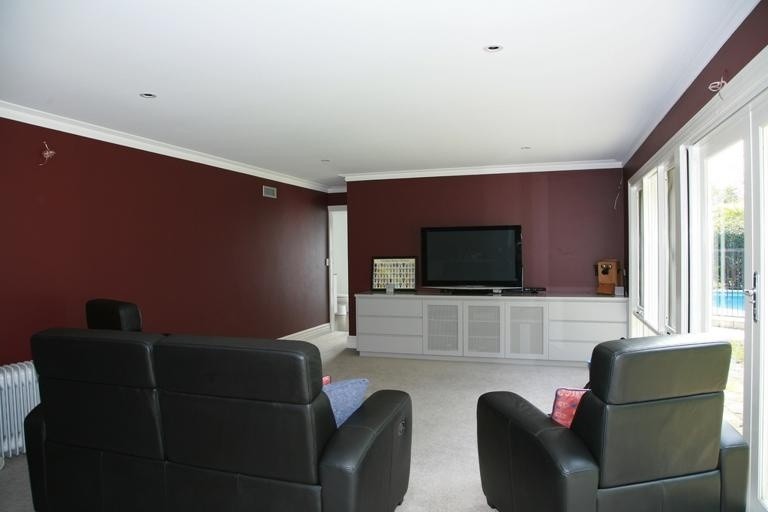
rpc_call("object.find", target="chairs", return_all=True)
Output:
[473,333,752,512]
[87,297,143,332]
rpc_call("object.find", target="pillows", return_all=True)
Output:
[550,385,591,428]
[320,377,371,427]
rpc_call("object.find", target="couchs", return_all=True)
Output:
[20,325,415,509]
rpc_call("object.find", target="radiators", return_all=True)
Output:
[2,360,39,472]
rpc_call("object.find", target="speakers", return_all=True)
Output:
[598,259,622,295]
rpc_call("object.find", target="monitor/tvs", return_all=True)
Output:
[370,256,417,292]
[420,225,522,296]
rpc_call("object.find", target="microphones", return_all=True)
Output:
[524,288,545,291]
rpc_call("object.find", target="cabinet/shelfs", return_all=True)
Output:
[353,290,630,363]
